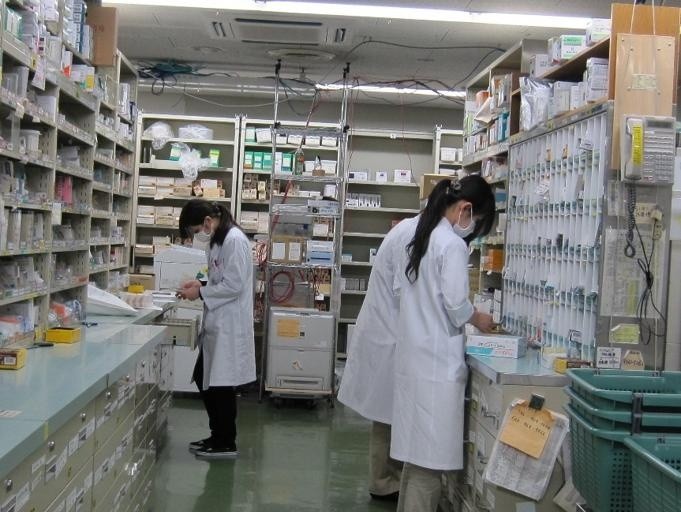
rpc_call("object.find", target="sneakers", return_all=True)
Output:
[189,438,239,456]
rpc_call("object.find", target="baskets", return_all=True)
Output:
[563,368,681,512]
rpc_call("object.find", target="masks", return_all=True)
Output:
[194,220,211,241]
[453,206,475,237]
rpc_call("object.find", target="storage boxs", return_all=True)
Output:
[418,173,459,199]
[272,234,302,264]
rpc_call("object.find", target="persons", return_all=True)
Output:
[175,198,257,459]
[336,210,423,503]
[389,174,499,512]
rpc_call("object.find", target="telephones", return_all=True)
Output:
[619,113,676,185]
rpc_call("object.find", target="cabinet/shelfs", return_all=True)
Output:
[345,126,440,360]
[240,117,344,337]
[141,113,239,293]
